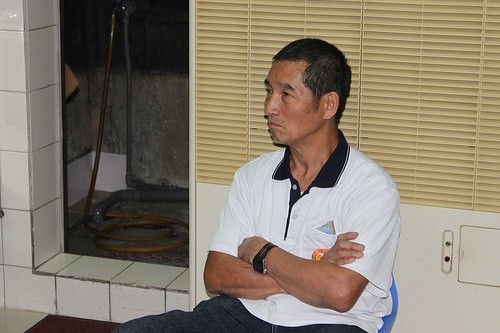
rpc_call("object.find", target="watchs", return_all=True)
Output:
[252,242,279,275]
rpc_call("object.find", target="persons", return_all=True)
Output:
[119,39,401,333]
